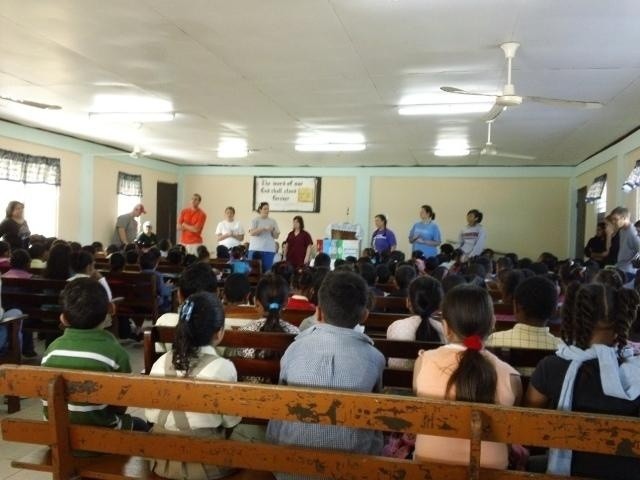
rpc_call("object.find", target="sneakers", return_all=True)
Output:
[21,353,41,360]
[130,326,143,342]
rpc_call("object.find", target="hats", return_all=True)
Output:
[144,221,152,227]
[135,204,146,213]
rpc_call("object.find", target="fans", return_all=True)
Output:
[446,118,537,162]
[93,127,176,160]
[440,41,605,125]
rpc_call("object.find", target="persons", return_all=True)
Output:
[0,201,30,242]
[583,207,640,288]
[408,205,442,258]
[177,194,207,256]
[281,215,313,270]
[459,209,487,257]
[38,263,640,480]
[216,207,245,253]
[369,214,396,253]
[247,202,280,271]
[110,204,147,248]
[136,220,158,249]
[1,234,640,382]
